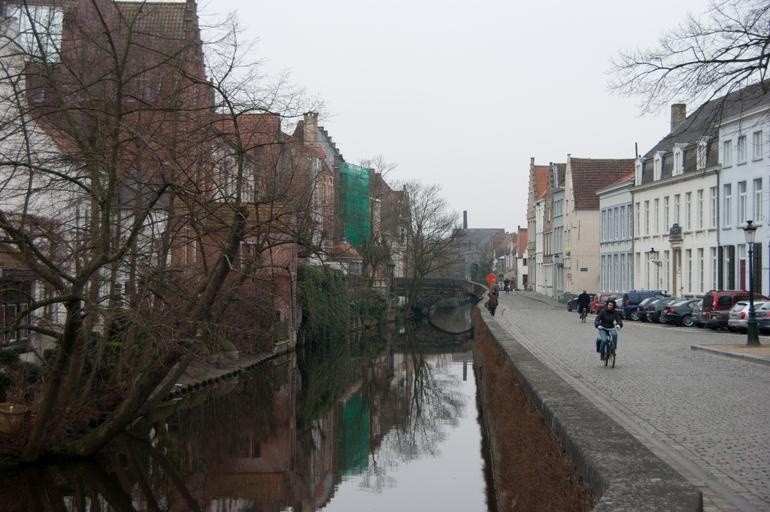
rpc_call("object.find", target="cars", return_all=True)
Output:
[567,290,770,336]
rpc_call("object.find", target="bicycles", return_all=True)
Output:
[597,327,617,368]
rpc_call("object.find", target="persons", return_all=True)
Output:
[504,278,510,295]
[577,290,590,319]
[485,289,498,317]
[493,282,500,296]
[595,300,623,360]
[486,272,496,289]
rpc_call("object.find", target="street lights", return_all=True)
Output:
[742,219,759,345]
